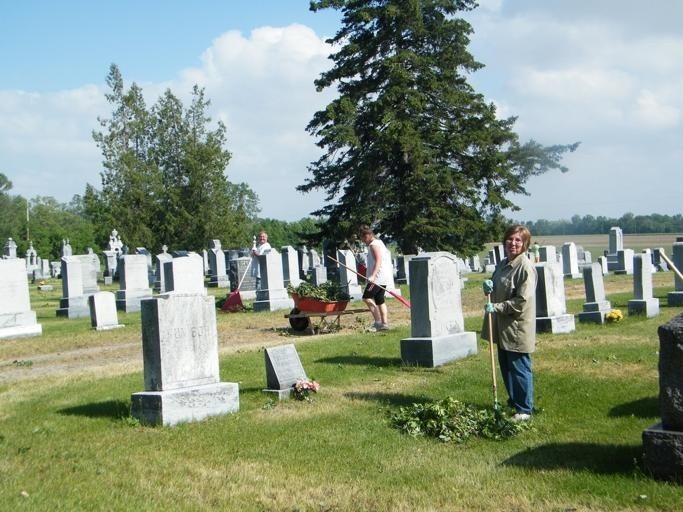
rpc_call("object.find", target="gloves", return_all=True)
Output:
[483,280,493,293]
[485,304,494,313]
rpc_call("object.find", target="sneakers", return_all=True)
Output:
[367,323,388,332]
[513,413,530,420]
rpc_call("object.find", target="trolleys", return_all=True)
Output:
[284,290,369,330]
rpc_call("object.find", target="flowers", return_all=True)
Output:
[293,378,320,401]
[605,308,622,323]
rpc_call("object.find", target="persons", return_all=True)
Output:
[357,224,393,332]
[249,231,272,298]
[481,224,539,421]
[531,241,540,263]
